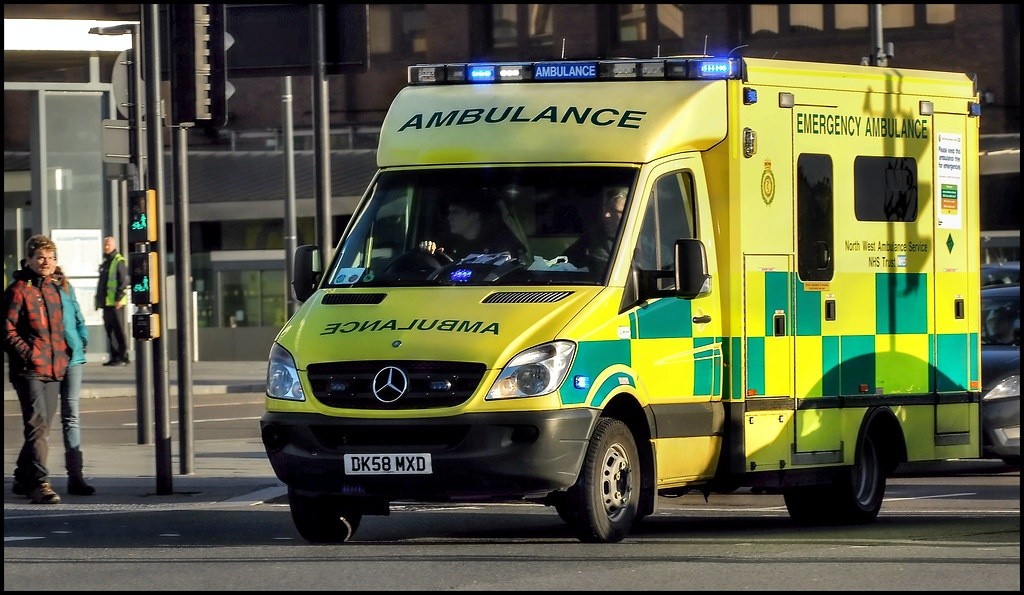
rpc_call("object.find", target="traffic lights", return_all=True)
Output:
[177,1,236,134]
[128,253,156,303]
[129,190,155,243]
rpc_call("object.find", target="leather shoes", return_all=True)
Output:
[103,359,115,366]
[111,360,130,366]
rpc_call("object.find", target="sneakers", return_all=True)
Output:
[12,478,32,496]
[31,483,62,504]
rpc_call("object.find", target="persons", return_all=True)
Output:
[95,236,130,367]
[560,186,675,304]
[50,253,95,495]
[3,235,74,503]
[417,198,517,265]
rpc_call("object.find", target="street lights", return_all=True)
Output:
[91,23,173,494]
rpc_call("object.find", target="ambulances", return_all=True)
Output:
[260,53,984,542]
[194,227,300,330]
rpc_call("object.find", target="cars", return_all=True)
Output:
[981,286,1020,458]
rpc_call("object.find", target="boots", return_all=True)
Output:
[64,450,96,496]
